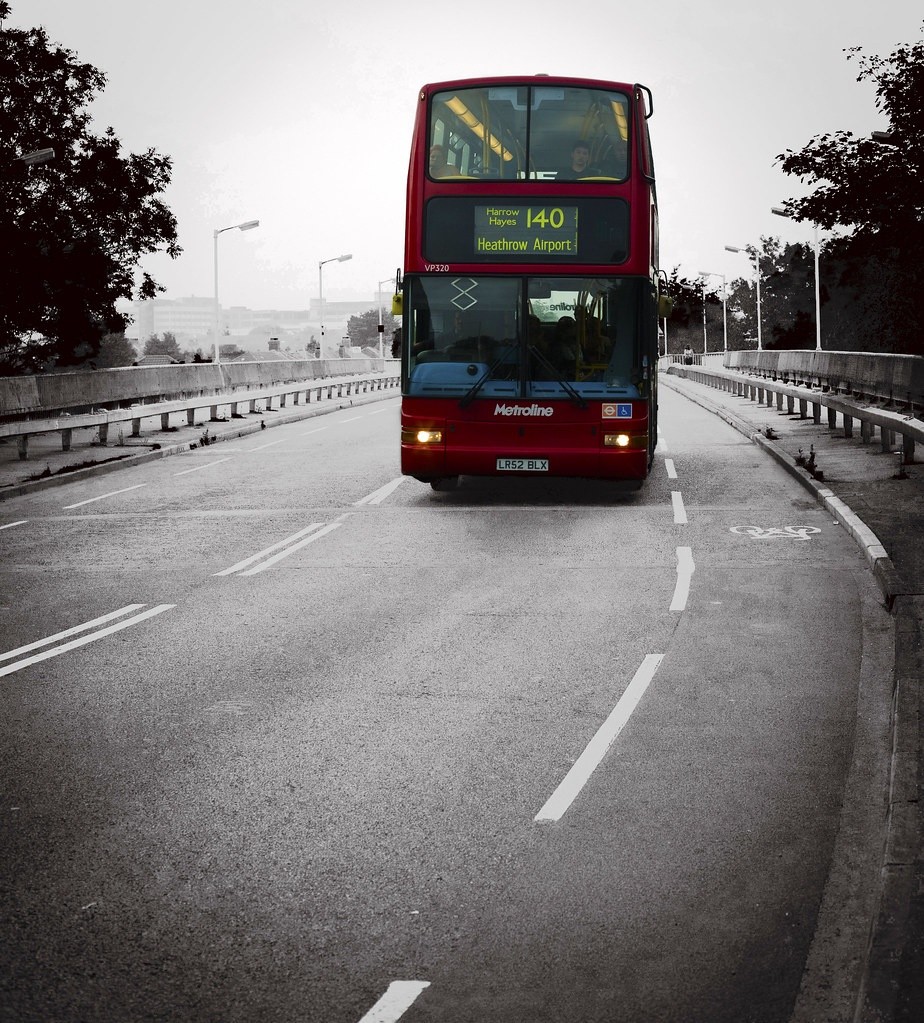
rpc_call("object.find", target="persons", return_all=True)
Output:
[429,144,452,178]
[556,141,599,181]
[591,142,627,177]
[528,307,612,383]
[684,344,693,365]
[441,310,486,350]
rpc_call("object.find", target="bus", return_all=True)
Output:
[392,73,675,494]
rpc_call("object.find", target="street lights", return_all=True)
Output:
[214,219,259,364]
[723,244,763,351]
[770,206,823,352]
[377,275,404,358]
[318,254,353,360]
[699,271,728,352]
